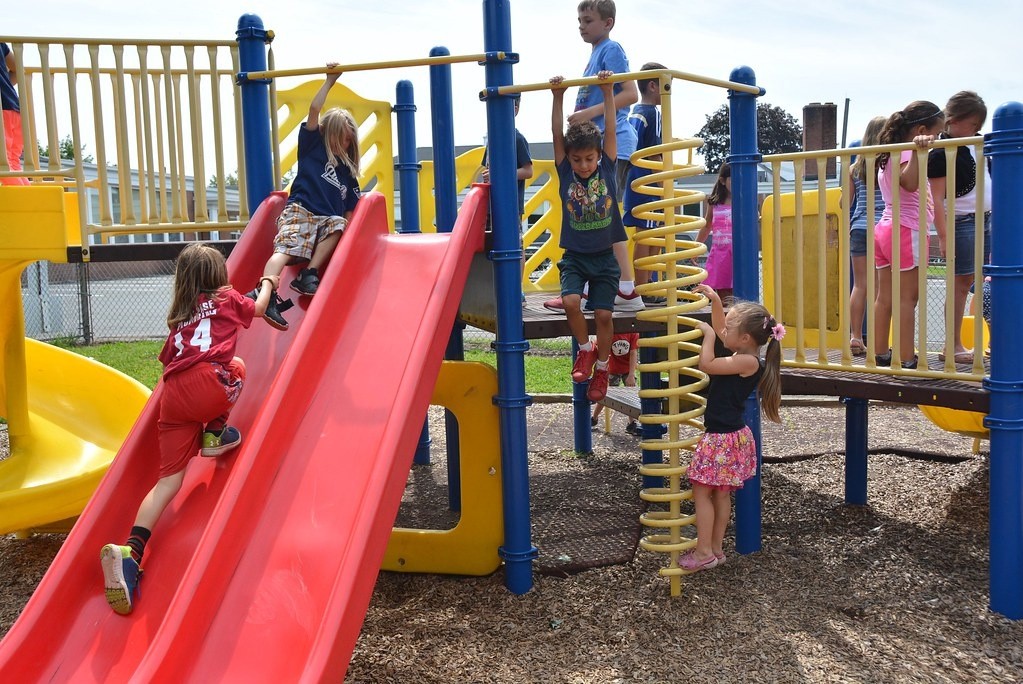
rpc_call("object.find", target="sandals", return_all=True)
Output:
[715,550,727,565]
[678,549,718,569]
[850,338,867,354]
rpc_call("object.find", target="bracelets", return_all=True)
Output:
[260,278,274,287]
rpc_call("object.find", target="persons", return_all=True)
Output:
[100,245,281,614]
[244,63,361,330]
[591,333,642,436]
[839,116,890,354]
[549,71,627,401]
[0,43,31,186]
[689,160,733,307]
[480,97,534,308]
[679,284,785,570]
[621,62,672,306]
[542,0,644,314]
[875,100,945,381]
[927,91,992,363]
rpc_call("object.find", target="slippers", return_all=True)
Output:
[938,350,974,363]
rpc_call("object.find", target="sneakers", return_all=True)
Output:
[543,291,594,313]
[625,420,643,435]
[571,341,598,383]
[892,354,942,380]
[634,278,667,306]
[876,348,893,366]
[590,416,599,425]
[288,271,319,295]
[100,543,144,614]
[201,423,241,457]
[587,364,610,402]
[614,288,646,311]
[245,288,290,332]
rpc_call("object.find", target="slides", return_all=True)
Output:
[0,186,153,538]
[1,184,486,684]
